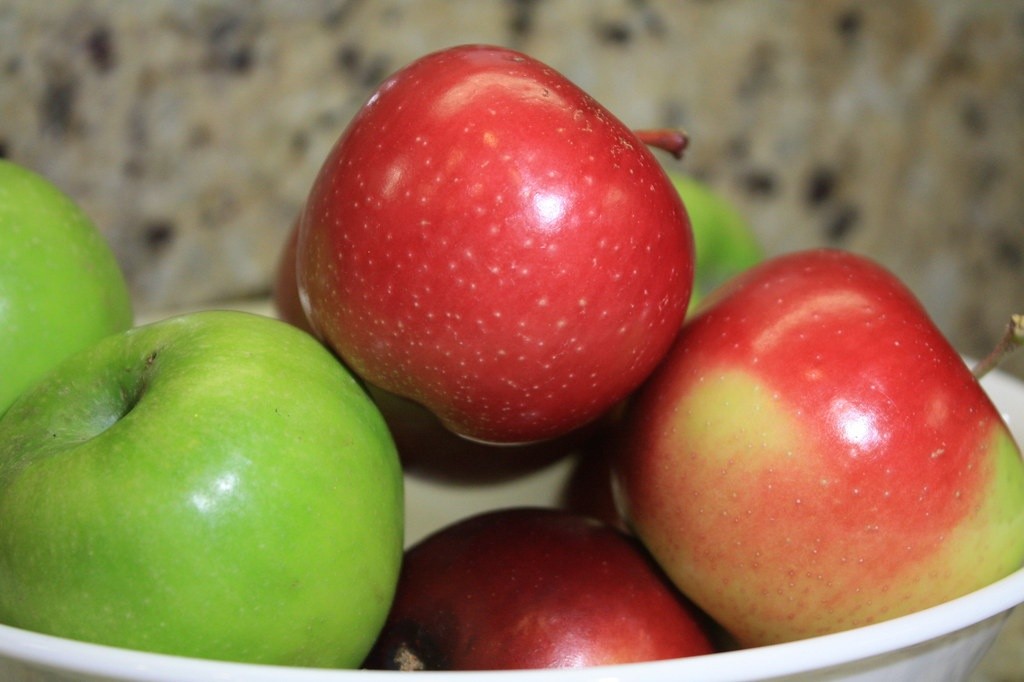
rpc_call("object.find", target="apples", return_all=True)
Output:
[0,41,1024,672]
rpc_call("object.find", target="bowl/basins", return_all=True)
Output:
[0,360,1024,682]
